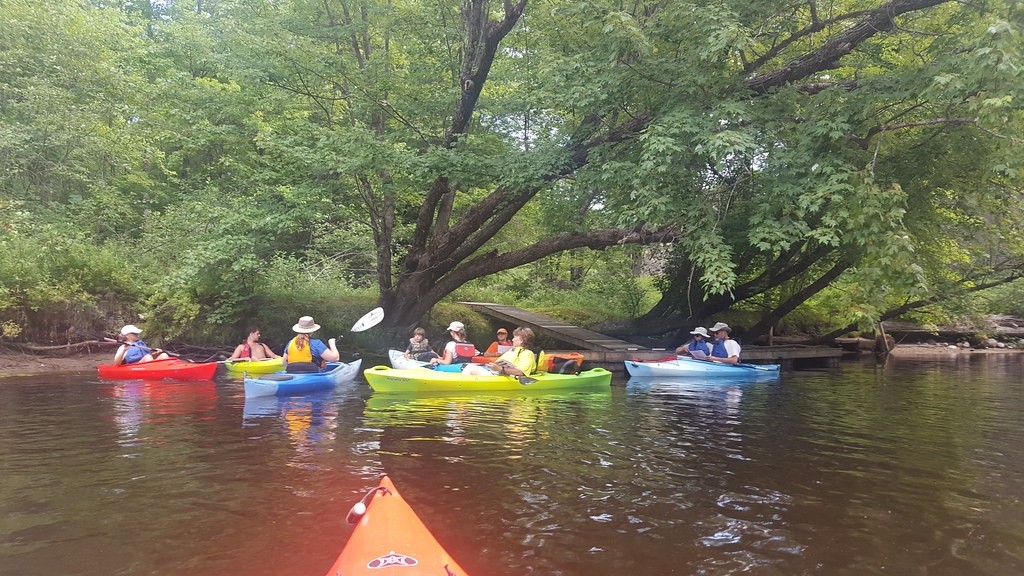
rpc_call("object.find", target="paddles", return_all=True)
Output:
[77,331,194,359]
[415,362,437,367]
[507,373,539,386]
[612,347,676,351]
[192,358,272,364]
[673,354,787,373]
[328,306,385,349]
[475,347,484,356]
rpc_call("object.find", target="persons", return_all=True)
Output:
[404,327,440,361]
[706,322,741,363]
[484,328,512,356]
[486,327,537,376]
[283,316,339,367]
[430,321,483,365]
[114,325,169,364]
[230,326,281,362]
[676,327,713,356]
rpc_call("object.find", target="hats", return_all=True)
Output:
[497,328,508,334]
[414,326,426,341]
[447,321,465,332]
[292,316,321,333]
[690,327,710,337]
[121,325,143,335]
[709,322,732,333]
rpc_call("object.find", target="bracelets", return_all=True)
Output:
[502,367,505,373]
